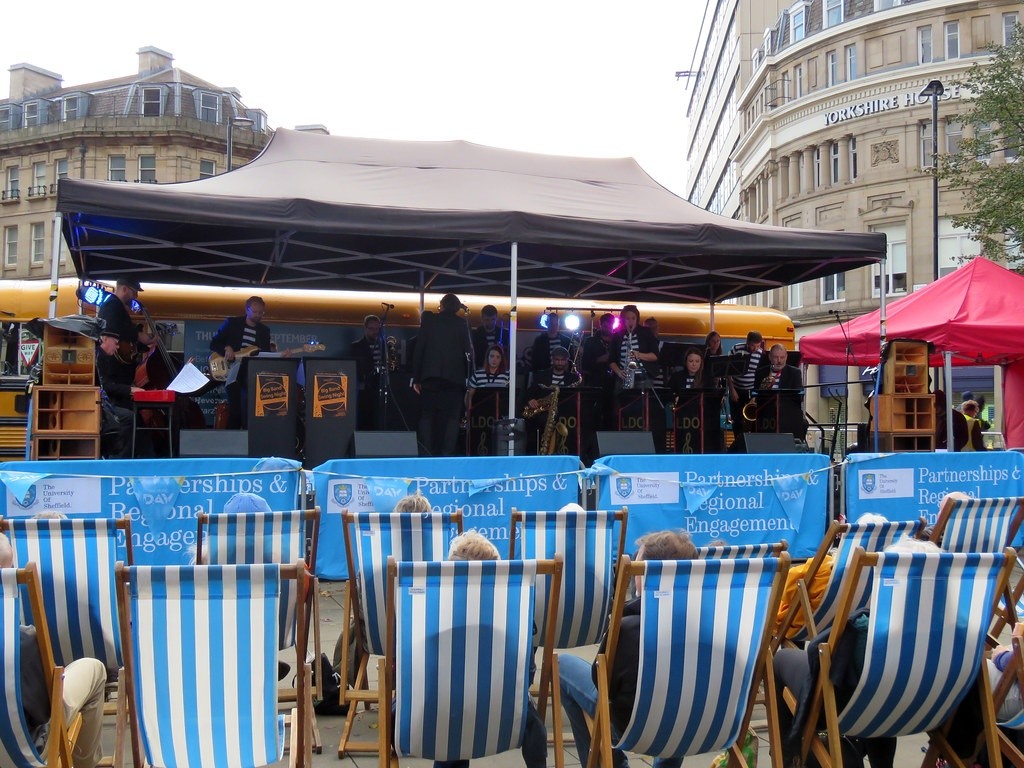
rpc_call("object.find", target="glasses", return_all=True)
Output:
[248,307,265,315]
[125,284,137,294]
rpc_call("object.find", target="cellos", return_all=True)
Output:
[128,299,206,458]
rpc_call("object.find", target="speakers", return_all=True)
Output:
[341,430,419,457]
[591,430,656,459]
[177,428,249,458]
[726,431,797,454]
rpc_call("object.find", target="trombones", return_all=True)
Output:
[567,328,583,372]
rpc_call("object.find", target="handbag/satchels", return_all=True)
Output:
[710,731,759,768]
[293,654,351,716]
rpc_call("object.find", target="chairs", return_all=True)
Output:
[0,497,1024,768]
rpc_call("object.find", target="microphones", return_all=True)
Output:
[381,302,394,309]
[461,303,468,311]
[828,309,846,315]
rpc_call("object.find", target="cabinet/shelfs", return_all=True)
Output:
[30,323,101,461]
[869,341,936,453]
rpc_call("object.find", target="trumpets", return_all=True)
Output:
[722,396,734,425]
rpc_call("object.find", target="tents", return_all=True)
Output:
[49,127,887,456]
[800,256,1024,451]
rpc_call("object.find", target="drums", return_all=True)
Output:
[296,417,306,457]
[214,399,228,429]
[296,383,306,417]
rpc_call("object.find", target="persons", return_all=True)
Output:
[189,493,291,681]
[921,622,1024,758]
[933,390,991,452]
[557,492,972,768]
[97,274,809,458]
[559,503,585,512]
[0,512,114,768]
[391,529,537,768]
[333,495,432,689]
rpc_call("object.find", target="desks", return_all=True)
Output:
[131,403,176,459]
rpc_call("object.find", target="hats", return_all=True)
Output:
[551,347,571,360]
[102,327,121,340]
[224,493,273,512]
[116,273,144,292]
[962,391,976,401]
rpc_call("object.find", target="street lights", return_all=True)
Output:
[922,80,945,389]
[226,116,255,172]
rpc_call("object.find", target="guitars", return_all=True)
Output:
[114,323,177,367]
[208,341,326,382]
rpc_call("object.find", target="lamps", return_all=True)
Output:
[76,278,114,306]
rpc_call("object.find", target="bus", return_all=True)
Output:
[1,279,795,456]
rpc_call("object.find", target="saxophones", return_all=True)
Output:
[743,364,774,429]
[523,360,583,418]
[523,384,567,456]
[386,335,400,372]
[622,329,639,389]
